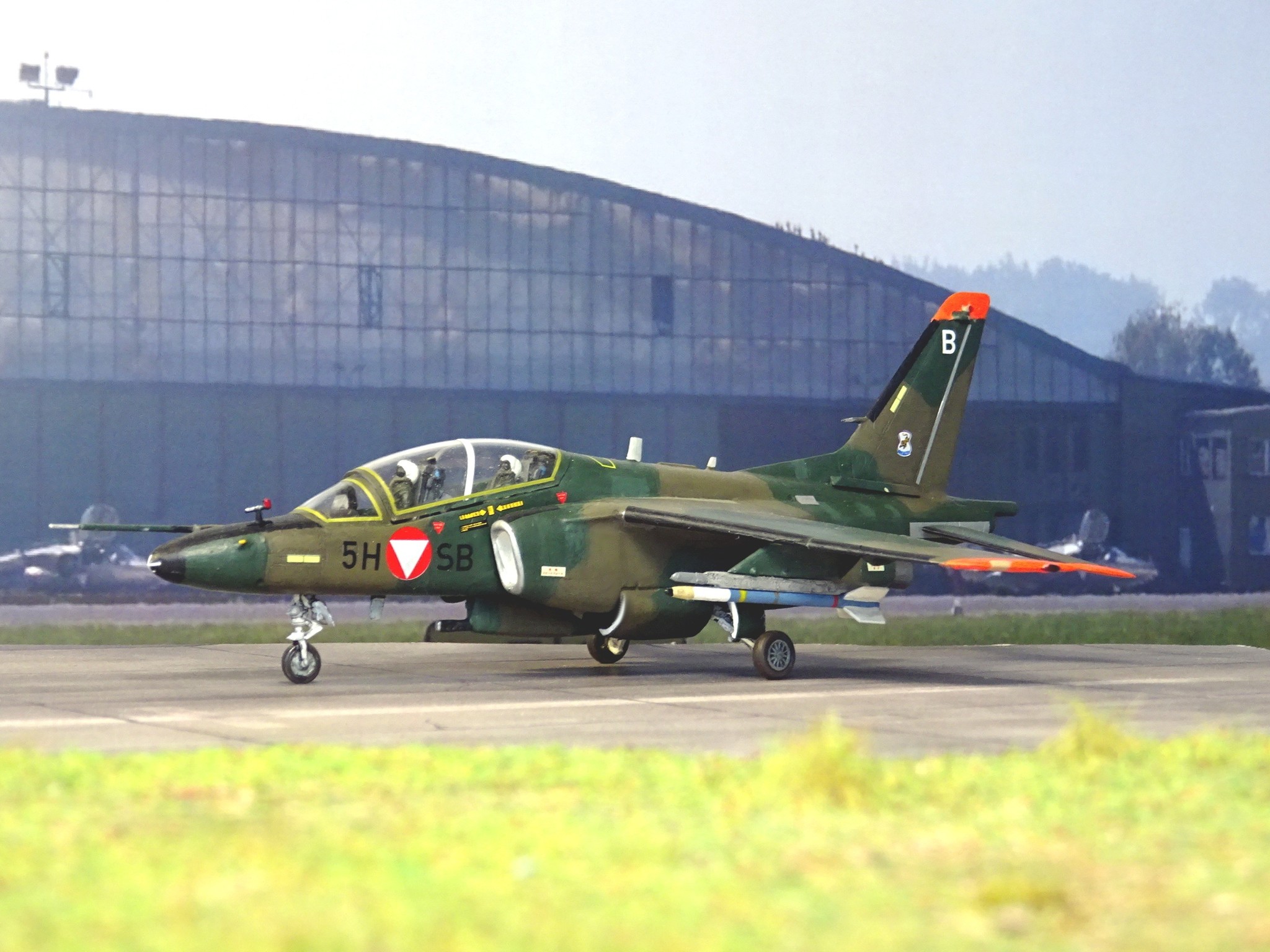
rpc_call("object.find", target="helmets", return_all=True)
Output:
[396,460,420,485]
[500,455,522,479]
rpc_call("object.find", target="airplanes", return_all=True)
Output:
[49,292,1138,685]
[926,509,1159,616]
[0,504,175,595]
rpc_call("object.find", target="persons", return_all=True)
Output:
[388,460,420,510]
[487,455,522,491]
